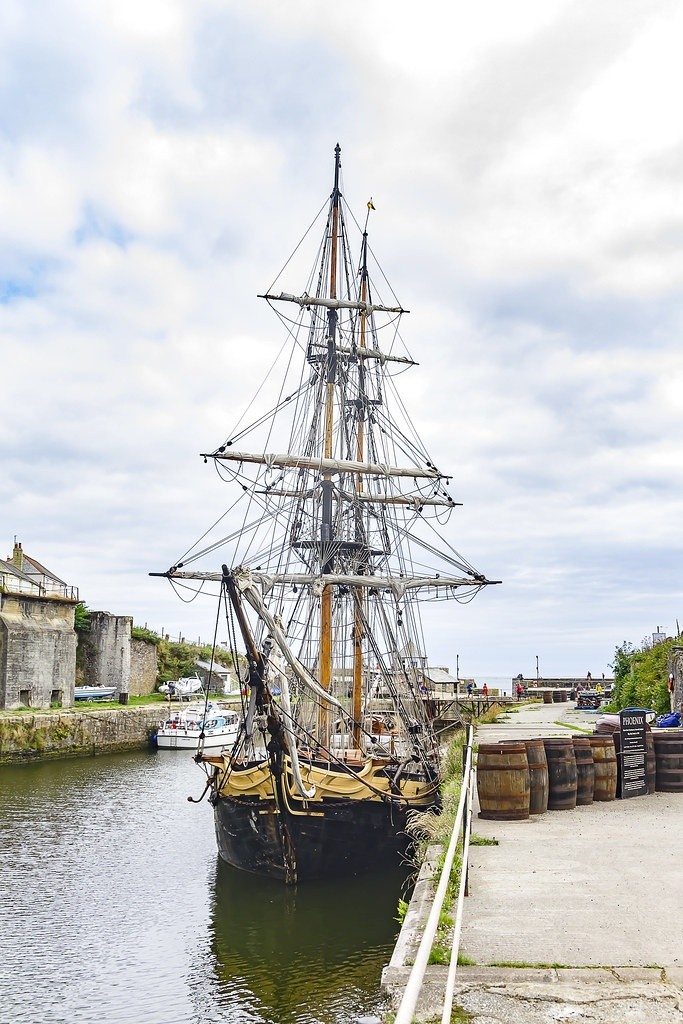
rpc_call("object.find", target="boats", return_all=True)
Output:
[617,707,658,724]
[74,685,117,700]
[158,675,204,693]
[147,670,241,750]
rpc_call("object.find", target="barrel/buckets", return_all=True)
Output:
[572,734,617,801]
[476,744,531,820]
[572,738,595,805]
[119,693,128,705]
[553,691,562,703]
[531,738,578,810]
[499,740,549,814]
[544,691,552,703]
[571,692,576,700]
[652,732,683,793]
[613,732,656,794]
[561,690,567,702]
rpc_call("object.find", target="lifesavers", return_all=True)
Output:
[668,674,674,693]
[187,721,196,730]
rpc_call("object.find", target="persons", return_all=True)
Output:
[576,671,605,696]
[160,714,179,731]
[375,663,380,669]
[466,682,487,698]
[516,671,538,702]
[209,720,215,728]
[427,688,433,700]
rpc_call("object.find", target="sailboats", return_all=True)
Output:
[147,145,503,892]
